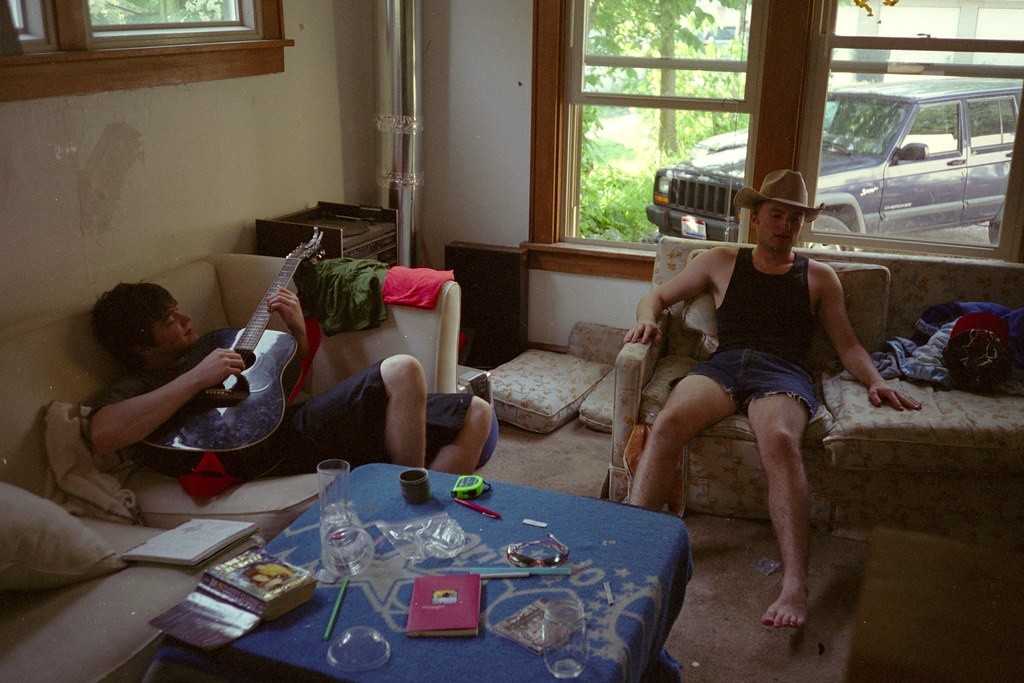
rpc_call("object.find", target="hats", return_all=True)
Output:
[733,168,826,222]
[942,311,1013,394]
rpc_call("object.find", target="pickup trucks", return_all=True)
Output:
[645,77,1024,252]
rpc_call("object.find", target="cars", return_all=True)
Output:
[695,24,739,52]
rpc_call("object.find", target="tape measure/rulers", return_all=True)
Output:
[450,475,491,498]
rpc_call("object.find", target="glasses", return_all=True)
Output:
[505,533,569,569]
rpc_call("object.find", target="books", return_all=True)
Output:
[406,573,484,636]
[148,545,320,652]
[119,519,261,576]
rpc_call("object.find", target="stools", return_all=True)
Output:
[789,531,1024,683]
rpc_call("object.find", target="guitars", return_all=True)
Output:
[138,223,325,483]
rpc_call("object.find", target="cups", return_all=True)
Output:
[317,457,351,524]
[318,504,375,577]
[397,469,430,505]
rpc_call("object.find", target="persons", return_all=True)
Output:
[87,282,493,476]
[622,169,923,628]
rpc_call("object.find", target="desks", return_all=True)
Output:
[146,462,693,683]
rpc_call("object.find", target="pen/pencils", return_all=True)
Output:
[479,572,531,578]
[323,576,349,640]
[454,498,502,519]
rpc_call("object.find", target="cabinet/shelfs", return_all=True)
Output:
[255,201,399,270]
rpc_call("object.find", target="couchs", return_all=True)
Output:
[0,254,461,683]
[599,234,1024,551]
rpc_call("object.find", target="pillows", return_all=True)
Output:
[0,482,126,591]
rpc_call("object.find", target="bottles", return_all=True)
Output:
[542,597,590,679]
